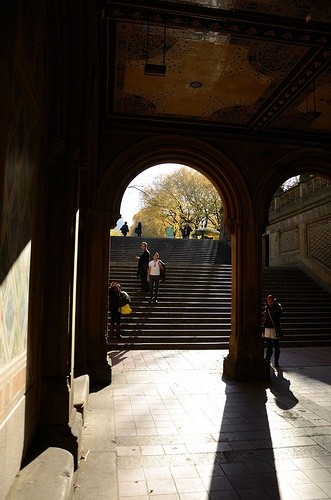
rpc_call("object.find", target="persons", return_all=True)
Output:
[263,294,285,368]
[182,224,193,240]
[120,222,130,237]
[146,251,166,306]
[136,241,151,297]
[107,281,131,340]
[135,222,143,237]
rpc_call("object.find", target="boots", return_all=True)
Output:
[108,324,114,337]
[116,325,120,338]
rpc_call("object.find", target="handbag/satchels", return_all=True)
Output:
[273,326,280,338]
[118,303,132,315]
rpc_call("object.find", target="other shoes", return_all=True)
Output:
[150,301,154,303]
[142,289,146,292]
[146,289,149,292]
[155,300,157,303]
[274,361,279,368]
[264,362,270,367]
